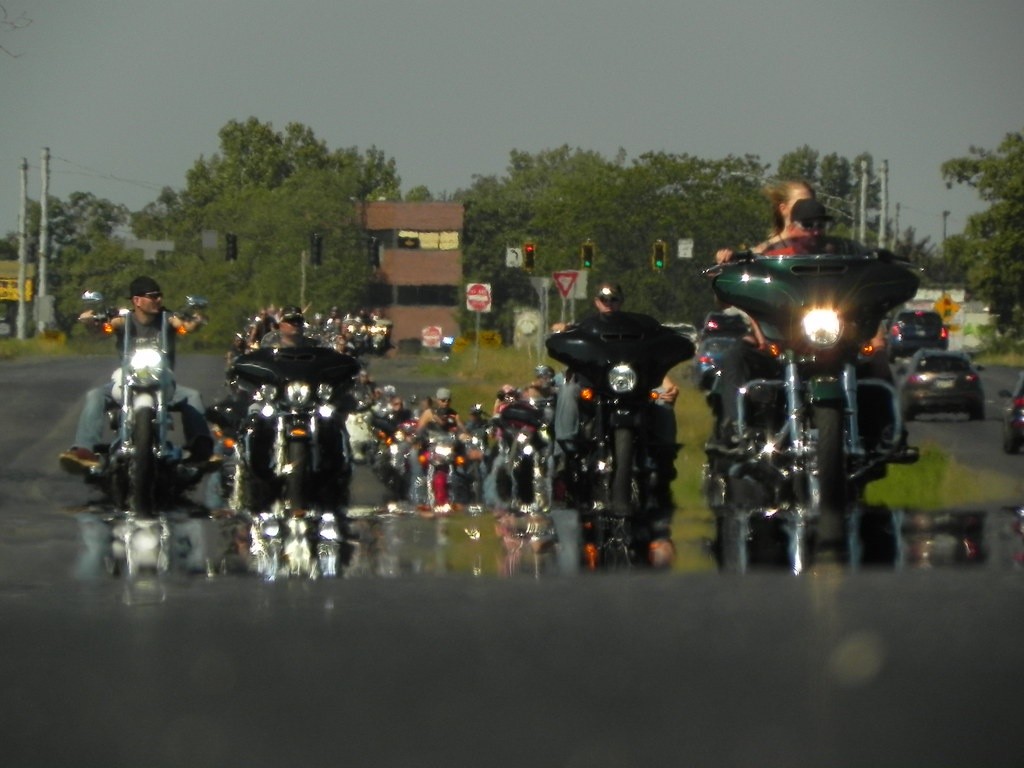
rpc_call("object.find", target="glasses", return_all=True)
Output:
[136,292,164,299]
[439,397,451,403]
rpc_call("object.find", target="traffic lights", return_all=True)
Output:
[652,241,666,271]
[524,242,535,271]
[580,242,595,272]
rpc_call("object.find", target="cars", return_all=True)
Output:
[888,308,949,364]
[898,348,986,421]
[997,371,1024,454]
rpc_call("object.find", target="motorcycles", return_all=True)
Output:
[212,328,372,511]
[344,366,558,518]
[76,287,220,514]
[701,237,927,574]
[223,299,396,362]
[541,312,698,516]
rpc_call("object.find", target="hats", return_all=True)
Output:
[791,198,832,220]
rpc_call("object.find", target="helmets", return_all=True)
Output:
[227,301,383,336]
[535,365,556,380]
[436,388,452,400]
[129,276,160,296]
[593,282,624,304]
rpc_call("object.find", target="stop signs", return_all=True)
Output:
[466,283,492,313]
[422,326,443,348]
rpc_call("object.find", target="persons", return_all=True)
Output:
[62,273,223,474]
[60,173,922,576]
[261,305,327,348]
[553,281,679,492]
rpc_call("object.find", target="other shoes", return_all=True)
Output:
[185,442,222,469]
[879,440,920,464]
[60,448,101,472]
